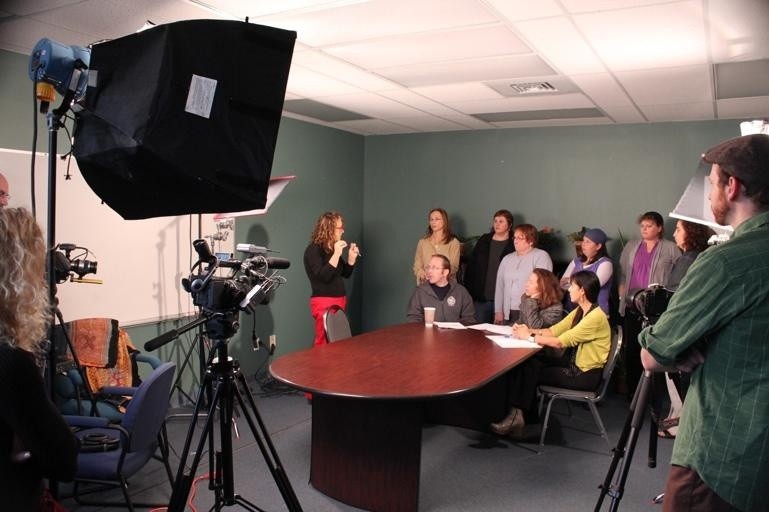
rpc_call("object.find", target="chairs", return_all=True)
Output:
[60,362,176,512]
[323,304,352,343]
[536,325,623,458]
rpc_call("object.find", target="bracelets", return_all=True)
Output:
[540,329,542,336]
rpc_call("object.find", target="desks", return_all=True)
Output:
[269,321,545,512]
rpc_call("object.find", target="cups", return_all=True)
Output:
[424,307,435,328]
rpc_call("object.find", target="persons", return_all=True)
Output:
[559,228,614,320]
[640,133,769,512]
[490,271,612,436]
[494,224,553,324]
[618,211,682,413]
[304,211,360,403]
[464,210,515,322]
[513,268,563,330]
[0,208,80,512]
[657,219,710,439]
[0,173,11,208]
[407,254,476,323]
[412,208,460,285]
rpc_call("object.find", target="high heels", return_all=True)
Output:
[490,408,525,437]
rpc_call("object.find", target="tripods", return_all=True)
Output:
[166,341,309,510]
[593,366,688,512]
[46,301,103,435]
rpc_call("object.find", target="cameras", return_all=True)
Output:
[625,283,671,323]
[59,242,99,286]
[139,239,290,351]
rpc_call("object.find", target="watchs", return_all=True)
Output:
[528,333,536,342]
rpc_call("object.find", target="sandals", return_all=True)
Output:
[658,428,677,439]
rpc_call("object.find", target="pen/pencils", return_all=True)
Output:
[356,252,362,257]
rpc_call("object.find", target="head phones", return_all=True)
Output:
[179,254,220,293]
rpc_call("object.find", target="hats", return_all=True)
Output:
[703,134,769,184]
[584,229,612,244]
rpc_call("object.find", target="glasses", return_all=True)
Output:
[425,266,447,270]
[336,225,343,229]
